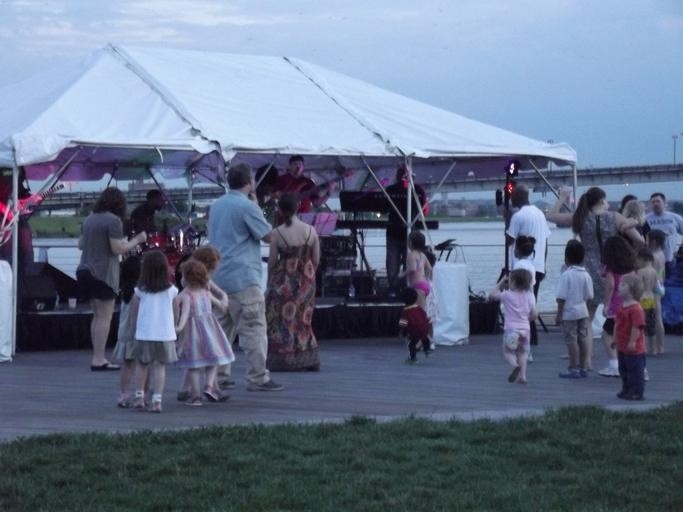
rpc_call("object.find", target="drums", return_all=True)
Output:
[147,229,197,284]
[318,235,357,268]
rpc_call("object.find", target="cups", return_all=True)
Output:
[67,298,77,309]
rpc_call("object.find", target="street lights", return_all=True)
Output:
[671,135,678,165]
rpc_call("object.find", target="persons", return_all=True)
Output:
[386,168,429,301]
[398,230,435,351]
[398,286,431,364]
[489,184,683,399]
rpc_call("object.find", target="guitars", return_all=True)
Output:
[264,170,356,212]
[1,184,65,225]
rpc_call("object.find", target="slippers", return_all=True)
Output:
[91,363,121,370]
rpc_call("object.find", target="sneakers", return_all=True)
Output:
[118,390,162,412]
[559,356,620,378]
[177,379,282,406]
[617,393,642,400]
